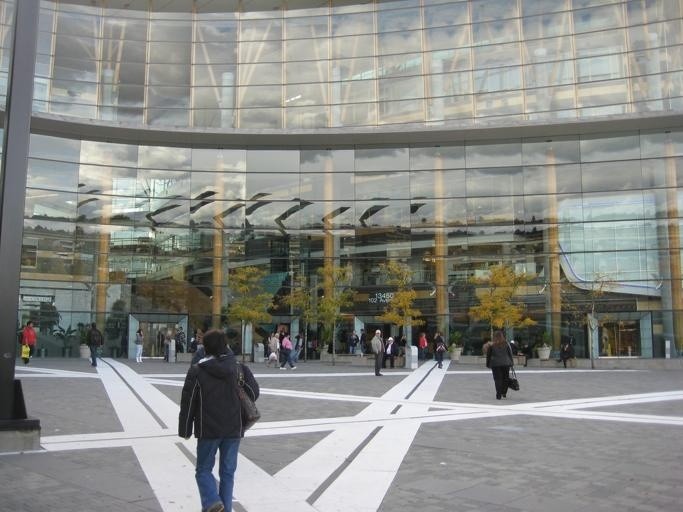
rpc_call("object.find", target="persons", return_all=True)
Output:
[21,321,37,366]
[485,331,575,400]
[348,329,449,376]
[135,329,145,362]
[179,331,259,512]
[86,323,103,367]
[174,327,234,367]
[263,331,317,370]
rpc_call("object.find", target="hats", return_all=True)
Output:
[375,329,381,334]
[387,337,393,341]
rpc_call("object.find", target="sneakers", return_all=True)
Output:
[267,364,297,370]
[377,374,382,376]
[200,500,225,512]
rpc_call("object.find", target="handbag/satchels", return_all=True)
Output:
[435,344,446,352]
[268,352,277,362]
[507,377,521,390]
[239,387,261,432]
[21,345,30,359]
